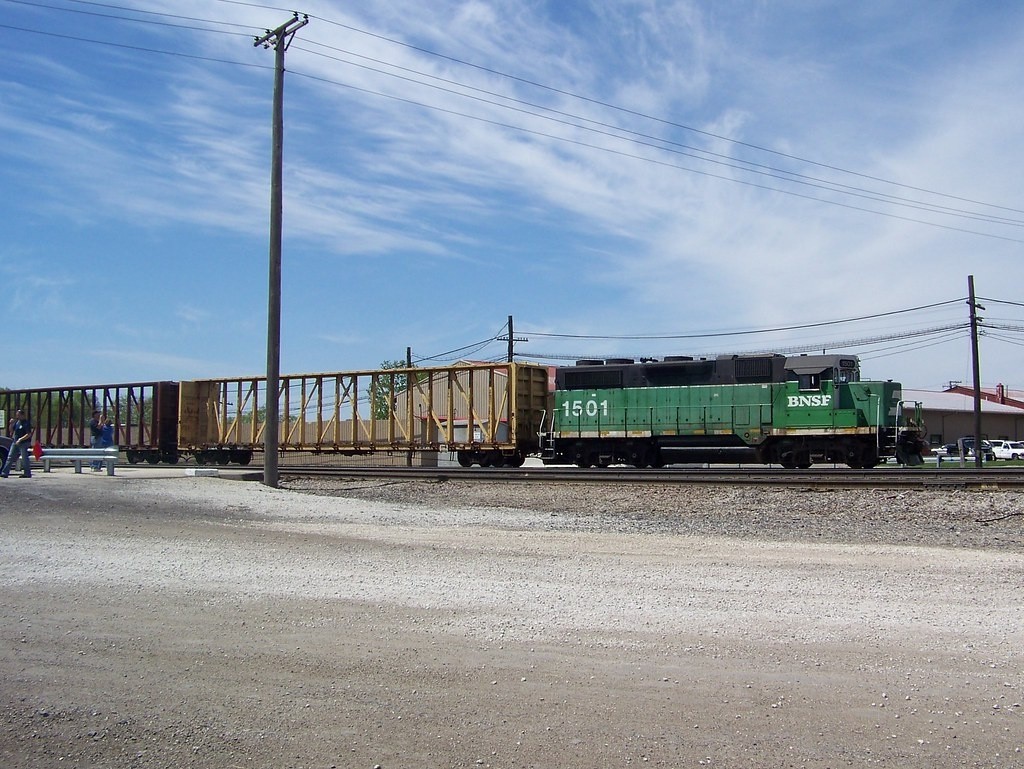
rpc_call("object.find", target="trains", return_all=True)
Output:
[0,352,930,470]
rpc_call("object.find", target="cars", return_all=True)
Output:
[930,444,968,456]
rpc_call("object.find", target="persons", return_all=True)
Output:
[0,410,32,478]
[89,411,113,472]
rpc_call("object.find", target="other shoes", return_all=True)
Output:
[0,474,8,478]
[90,467,103,473]
[19,475,31,478]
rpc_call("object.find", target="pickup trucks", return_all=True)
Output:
[945,436,997,462]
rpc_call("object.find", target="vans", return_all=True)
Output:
[988,439,1024,460]
